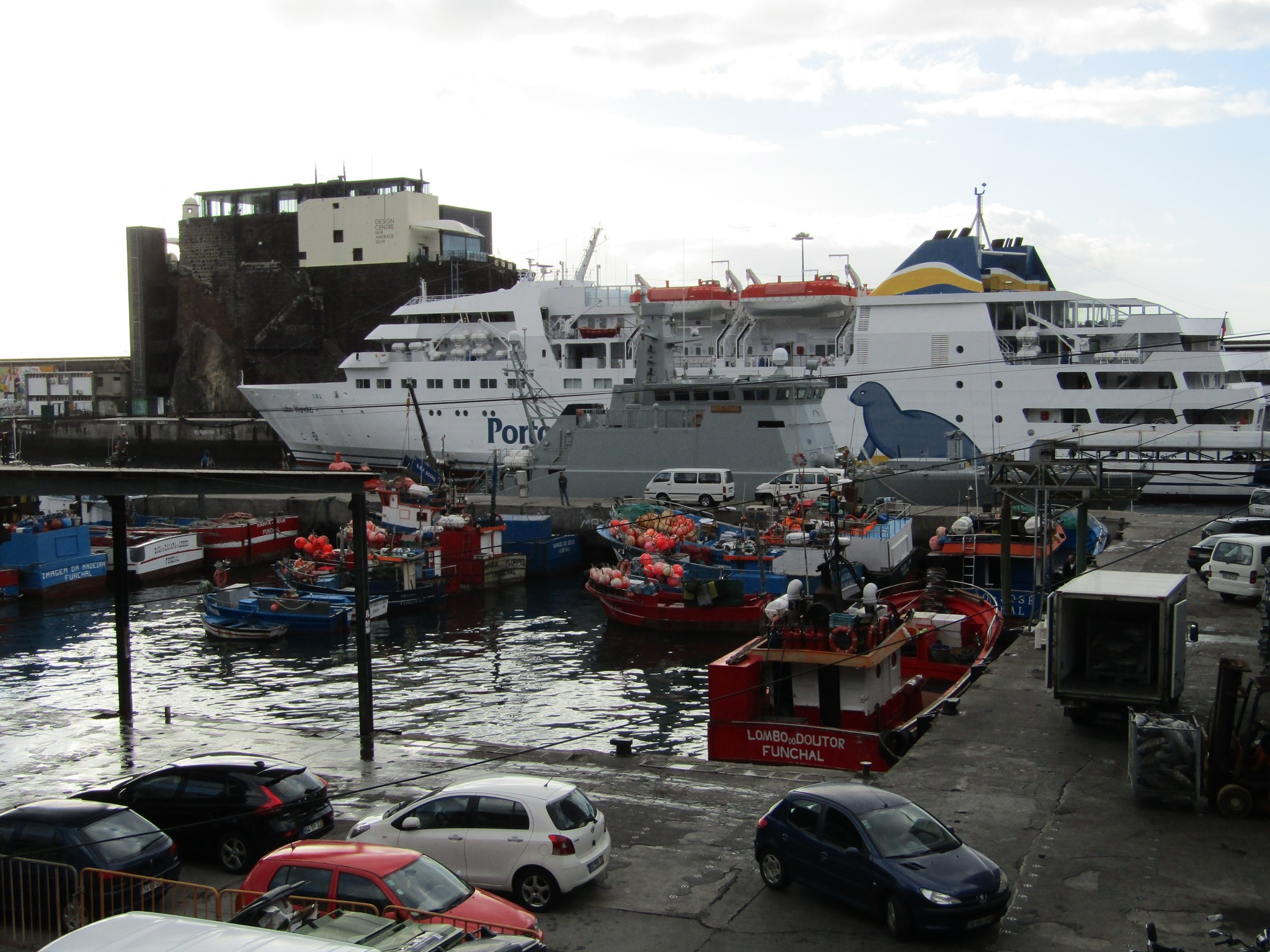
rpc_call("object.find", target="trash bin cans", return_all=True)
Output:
[41,405,54,417]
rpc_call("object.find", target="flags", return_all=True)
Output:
[1221,319,1226,341]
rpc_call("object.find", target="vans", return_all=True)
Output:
[644,468,735,507]
[1247,488,1270,517]
[1207,535,1270,607]
[755,468,846,507]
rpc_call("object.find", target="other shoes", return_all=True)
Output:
[562,504,565,506]
[567,504,570,506]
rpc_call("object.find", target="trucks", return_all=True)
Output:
[1046,570,1198,728]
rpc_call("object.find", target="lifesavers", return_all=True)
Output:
[462,514,473,526]
[741,540,757,556]
[495,512,504,523]
[693,413,703,427]
[214,568,226,586]
[829,626,858,654]
[844,515,858,521]
[866,625,878,651]
[788,521,802,532]
[620,559,631,577]
[769,523,784,540]
[794,452,806,465]
[860,513,866,521]
[819,356,827,364]
[879,617,890,643]
[393,476,404,489]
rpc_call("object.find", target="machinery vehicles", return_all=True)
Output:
[1128,655,1270,818]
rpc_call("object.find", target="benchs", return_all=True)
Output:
[227,623,246,628]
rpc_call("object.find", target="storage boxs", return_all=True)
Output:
[829,613,858,628]
[631,583,683,597]
[900,611,967,647]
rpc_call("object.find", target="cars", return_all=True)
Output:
[234,839,544,943]
[346,776,611,913]
[754,782,1012,942]
[1187,533,1261,585]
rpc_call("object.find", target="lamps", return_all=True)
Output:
[805,360,818,379]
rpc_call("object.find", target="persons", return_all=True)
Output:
[1063,554,1077,584]
[115,446,119,453]
[558,472,570,506]
[202,450,211,468]
[120,433,128,444]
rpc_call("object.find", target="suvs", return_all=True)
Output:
[1201,515,1270,539]
[0,800,181,933]
[67,751,335,874]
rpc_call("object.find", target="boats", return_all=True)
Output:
[232,181,1270,522]
[629,284,739,319]
[271,502,526,614]
[737,280,856,315]
[376,435,580,582]
[586,344,1108,627]
[0,416,299,611]
[196,557,388,640]
[707,408,1004,772]
